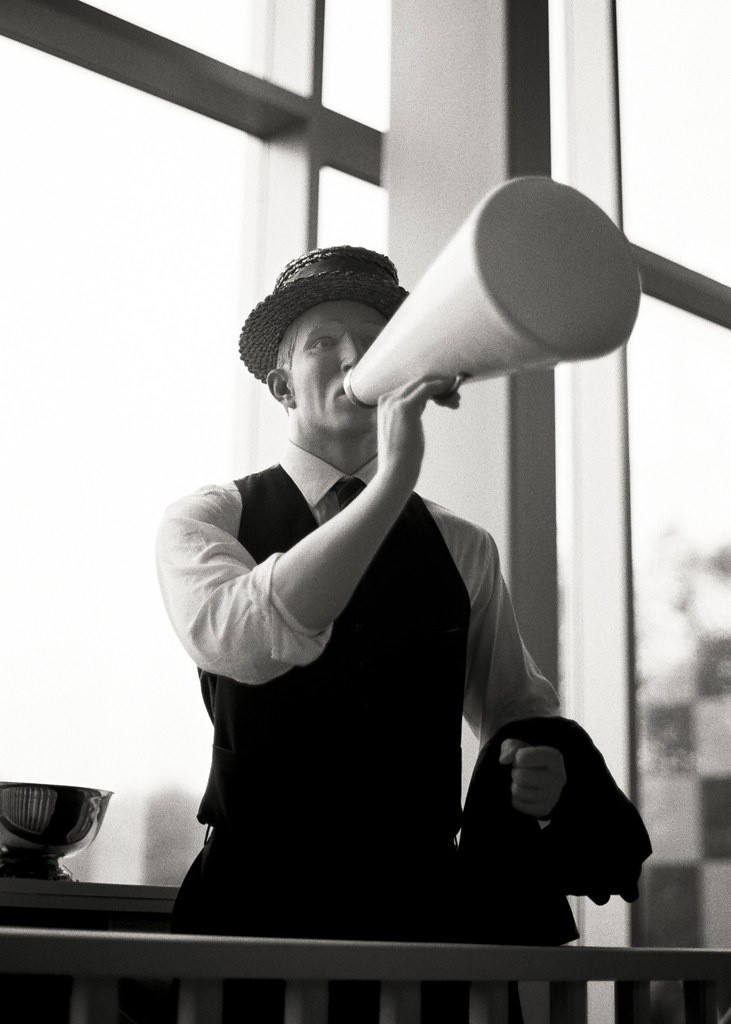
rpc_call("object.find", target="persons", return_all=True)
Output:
[149,246,566,1022]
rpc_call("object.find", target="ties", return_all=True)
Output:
[325,479,368,531]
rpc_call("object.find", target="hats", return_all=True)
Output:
[236,244,419,388]
